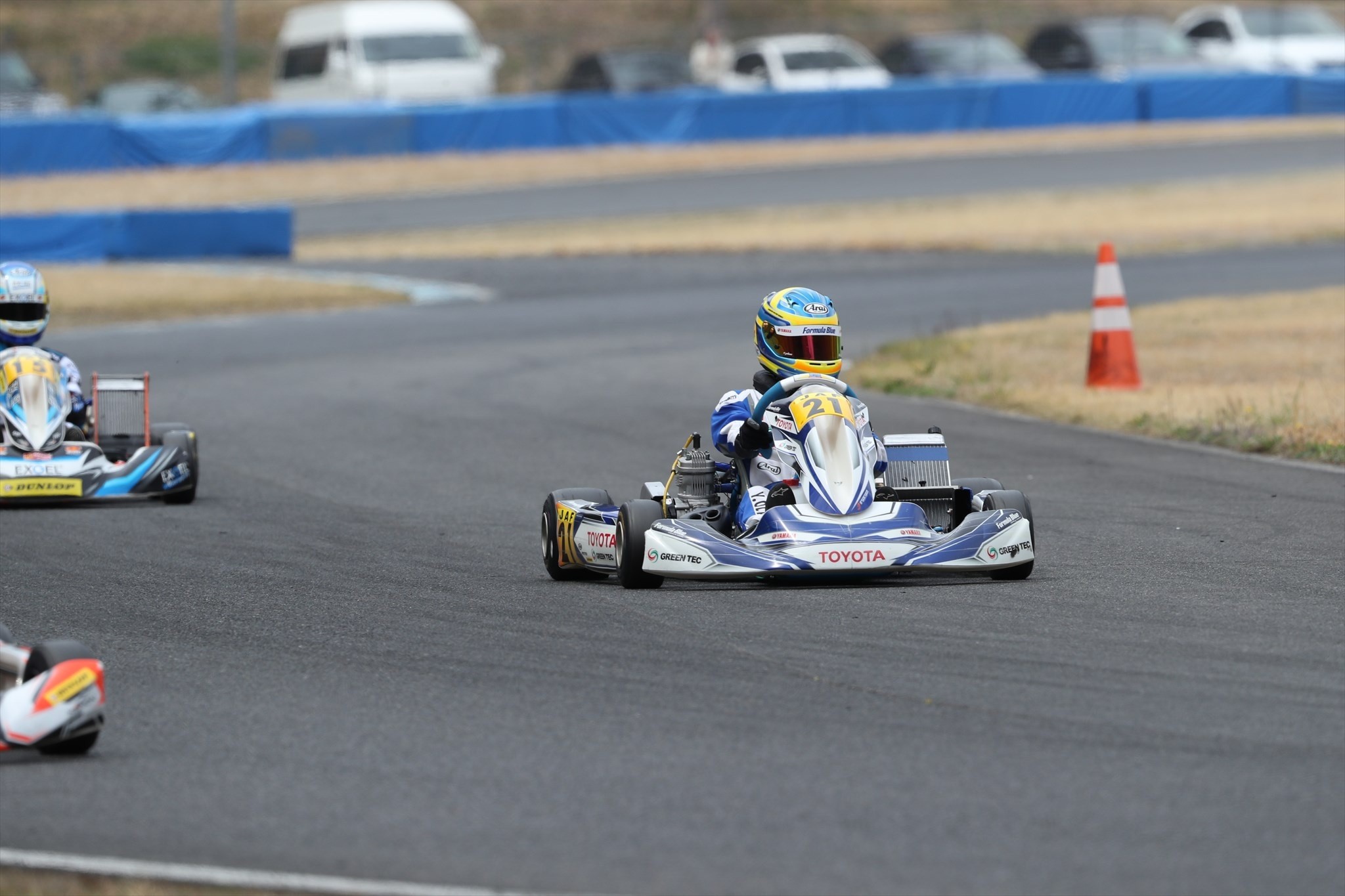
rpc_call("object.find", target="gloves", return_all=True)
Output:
[739,418,770,450]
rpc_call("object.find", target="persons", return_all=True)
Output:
[688,24,740,86]
[711,287,899,535]
[0,260,86,441]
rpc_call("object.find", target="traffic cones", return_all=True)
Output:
[1082,242,1151,392]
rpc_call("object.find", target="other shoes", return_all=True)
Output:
[764,478,797,510]
[874,484,896,503]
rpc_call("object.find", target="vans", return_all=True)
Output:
[272,0,510,113]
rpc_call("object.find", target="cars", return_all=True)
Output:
[686,33,894,90]
[877,26,1043,81]
[558,48,712,100]
[88,78,204,114]
[1027,16,1231,78]
[1167,7,1342,80]
[0,43,77,116]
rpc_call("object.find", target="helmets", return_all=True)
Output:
[752,286,842,380]
[0,260,50,347]
